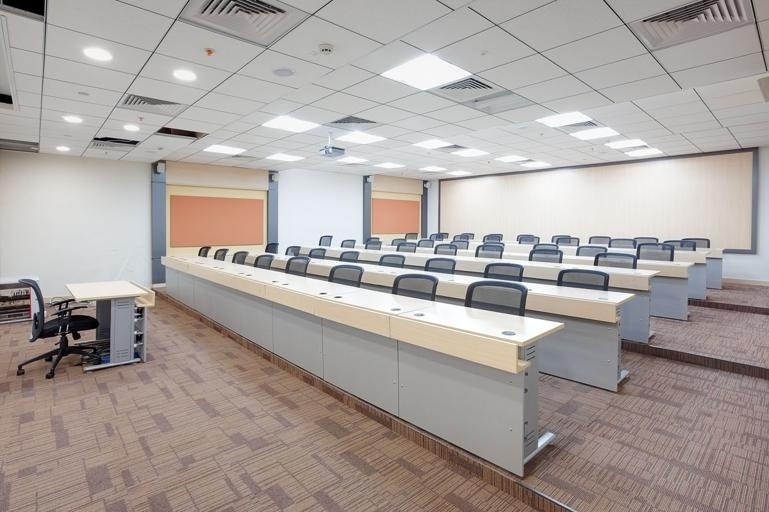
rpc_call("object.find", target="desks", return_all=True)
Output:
[66,280,156,374]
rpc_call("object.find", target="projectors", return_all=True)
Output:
[319,146,345,158]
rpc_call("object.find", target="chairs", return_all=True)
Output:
[16,278,100,381]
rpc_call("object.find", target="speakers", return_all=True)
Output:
[424,182,431,188]
[367,176,373,182]
[157,162,166,173]
[272,175,279,181]
